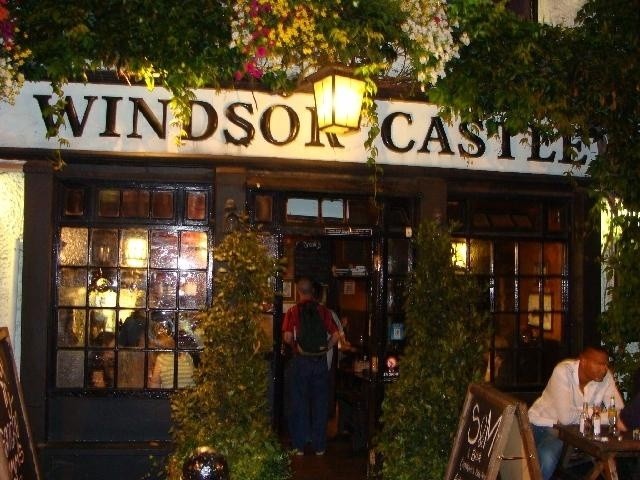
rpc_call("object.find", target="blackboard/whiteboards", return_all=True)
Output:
[443,384,516,480]
[0,327,40,480]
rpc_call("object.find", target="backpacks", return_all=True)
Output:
[293,301,329,358]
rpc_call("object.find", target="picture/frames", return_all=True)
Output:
[282,279,294,301]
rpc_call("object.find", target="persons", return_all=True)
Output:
[527,346,640,480]
[84,296,196,389]
[282,276,357,456]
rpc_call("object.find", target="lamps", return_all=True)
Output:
[306,63,367,135]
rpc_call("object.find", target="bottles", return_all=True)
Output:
[363,350,379,376]
[578,396,618,442]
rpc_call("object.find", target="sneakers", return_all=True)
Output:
[314,448,326,456]
[286,447,304,455]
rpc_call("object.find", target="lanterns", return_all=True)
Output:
[313,73,368,136]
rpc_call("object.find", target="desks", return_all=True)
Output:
[552,423,639,479]
